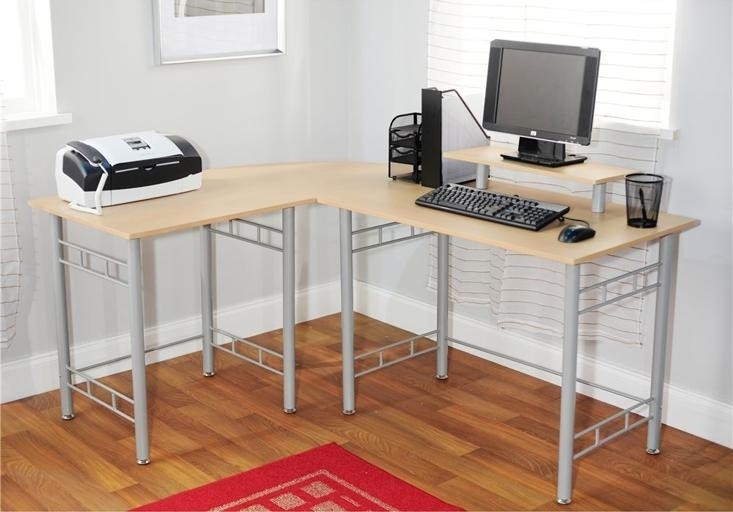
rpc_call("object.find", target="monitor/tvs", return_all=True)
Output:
[482,39,601,167]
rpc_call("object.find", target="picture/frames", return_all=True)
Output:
[152,0,286,66]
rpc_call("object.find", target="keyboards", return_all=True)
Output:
[415,182,570,231]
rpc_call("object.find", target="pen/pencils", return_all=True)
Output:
[637,187,648,224]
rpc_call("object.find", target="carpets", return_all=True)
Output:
[125,441,468,512]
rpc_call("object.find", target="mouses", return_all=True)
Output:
[558,225,596,242]
